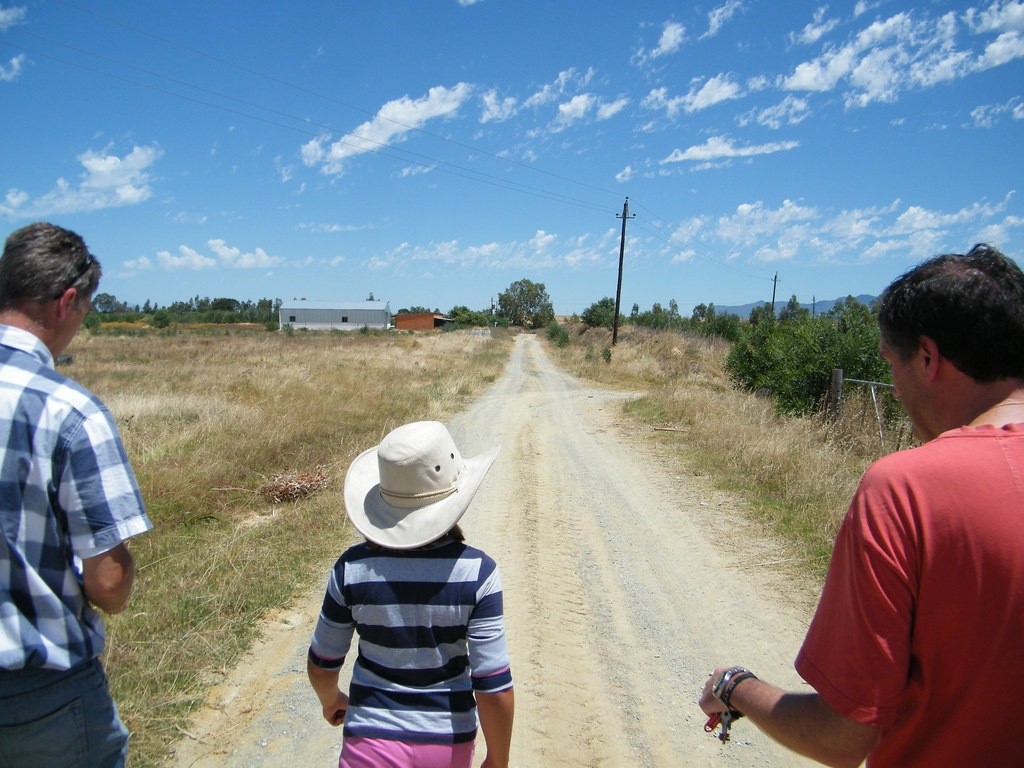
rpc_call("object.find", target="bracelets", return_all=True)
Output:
[711,667,757,710]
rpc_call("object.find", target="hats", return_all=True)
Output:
[344,419,502,550]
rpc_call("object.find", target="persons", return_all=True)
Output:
[0,221,155,768]
[306,420,515,768]
[698,241,1024,768]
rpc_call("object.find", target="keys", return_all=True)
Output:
[718,711,731,744]
